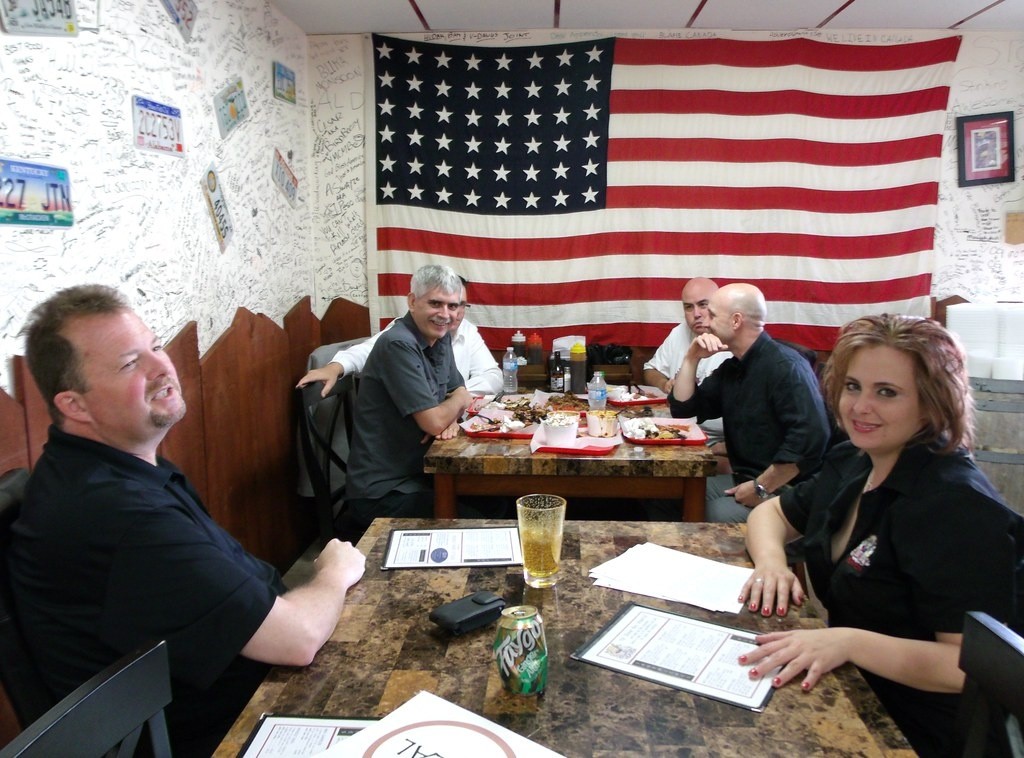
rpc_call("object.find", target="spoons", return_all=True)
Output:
[471,413,501,424]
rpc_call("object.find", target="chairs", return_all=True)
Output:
[298,377,383,546]
[943,610,1024,758]
[0,638,175,758]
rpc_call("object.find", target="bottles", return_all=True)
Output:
[570,344,586,395]
[551,350,564,393]
[564,367,571,392]
[502,347,518,392]
[587,370,607,411]
[511,330,527,367]
[527,333,544,364]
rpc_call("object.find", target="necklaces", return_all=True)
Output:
[867,482,873,490]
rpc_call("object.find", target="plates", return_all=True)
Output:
[603,372,634,381]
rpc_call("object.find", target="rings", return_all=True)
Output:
[755,578,763,582]
[447,428,453,431]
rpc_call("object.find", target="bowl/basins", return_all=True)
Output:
[592,364,629,374]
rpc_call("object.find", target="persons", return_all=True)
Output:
[344,265,486,527]
[296,275,504,398]
[643,277,734,474]
[667,281,830,522]
[1,285,366,758]
[738,314,1024,758]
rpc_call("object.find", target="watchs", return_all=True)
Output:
[753,480,768,498]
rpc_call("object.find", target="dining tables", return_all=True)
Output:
[421,386,719,522]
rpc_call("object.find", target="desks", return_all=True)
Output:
[211,518,919,758]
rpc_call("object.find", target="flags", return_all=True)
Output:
[364,30,963,350]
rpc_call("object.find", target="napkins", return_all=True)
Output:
[459,388,585,433]
[586,382,668,402]
[618,414,707,440]
[529,417,625,454]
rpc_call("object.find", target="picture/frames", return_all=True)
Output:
[955,110,1015,187]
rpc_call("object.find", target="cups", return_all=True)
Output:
[586,411,617,437]
[542,410,580,449]
[516,493,567,589]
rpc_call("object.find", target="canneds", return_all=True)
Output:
[493,606,547,696]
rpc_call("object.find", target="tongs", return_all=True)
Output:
[629,380,646,396]
